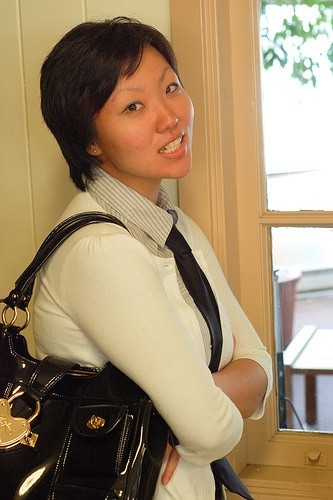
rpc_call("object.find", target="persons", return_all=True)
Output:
[30,16,273,500]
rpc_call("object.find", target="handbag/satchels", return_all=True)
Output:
[0,212,170,500]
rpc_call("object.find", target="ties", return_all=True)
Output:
[165,224,255,500]
